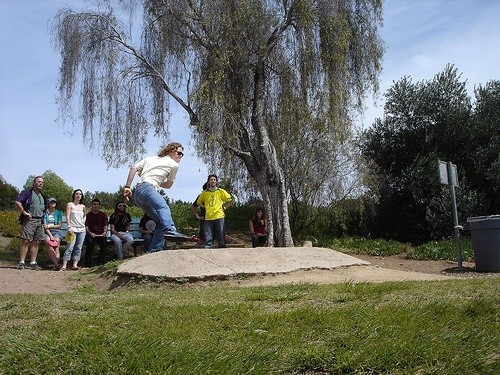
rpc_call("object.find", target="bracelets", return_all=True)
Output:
[67,226,71,228]
[103,230,107,233]
[223,206,227,211]
[125,186,131,189]
[126,231,129,233]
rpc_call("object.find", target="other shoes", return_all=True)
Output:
[163,231,189,242]
[17,262,25,269]
[29,263,43,271]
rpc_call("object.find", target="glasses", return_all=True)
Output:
[176,151,184,156]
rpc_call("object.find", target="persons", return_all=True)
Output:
[108,201,134,262]
[196,174,235,249]
[123,142,189,255]
[85,199,108,267]
[15,176,47,270]
[43,198,63,271]
[138,211,157,252]
[249,208,269,248]
[58,189,86,271]
[191,182,209,248]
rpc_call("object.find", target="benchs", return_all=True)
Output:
[42,215,189,268]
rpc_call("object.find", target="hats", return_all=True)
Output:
[48,198,56,203]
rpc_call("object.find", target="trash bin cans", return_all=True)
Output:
[467,215,500,272]
